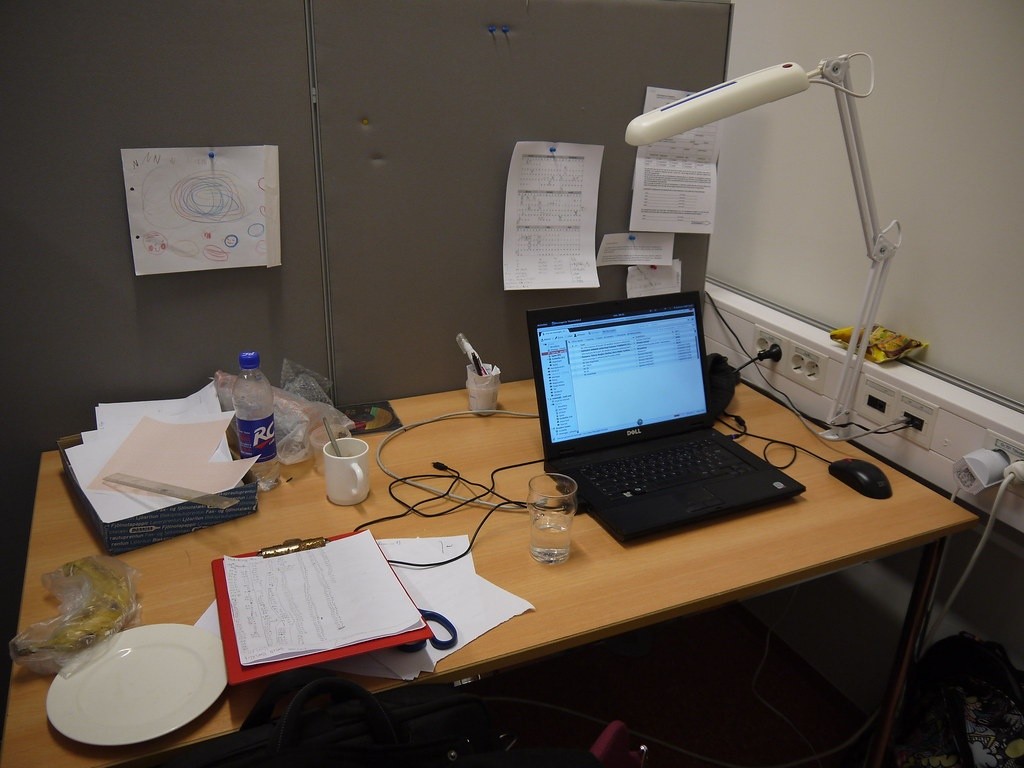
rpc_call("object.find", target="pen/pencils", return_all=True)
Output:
[456,333,488,376]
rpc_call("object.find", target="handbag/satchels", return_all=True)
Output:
[107,670,513,768]
[890,631,1024,768]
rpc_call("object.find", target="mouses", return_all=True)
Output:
[829,459,894,500]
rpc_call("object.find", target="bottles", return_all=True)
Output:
[231,350,281,492]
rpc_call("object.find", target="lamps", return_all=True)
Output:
[626,51,904,440]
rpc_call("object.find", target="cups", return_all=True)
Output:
[322,437,368,506]
[526,471,577,564]
[465,363,501,415]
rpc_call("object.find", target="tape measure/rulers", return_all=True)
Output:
[103,472,241,509]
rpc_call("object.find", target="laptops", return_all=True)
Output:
[524,289,806,542]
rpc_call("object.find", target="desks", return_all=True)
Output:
[0,365,981,768]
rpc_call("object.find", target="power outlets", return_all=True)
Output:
[980,426,1024,500]
[751,324,830,395]
[858,373,939,451]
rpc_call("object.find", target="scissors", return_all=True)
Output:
[396,608,458,653]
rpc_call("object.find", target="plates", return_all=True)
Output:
[45,623,227,745]
